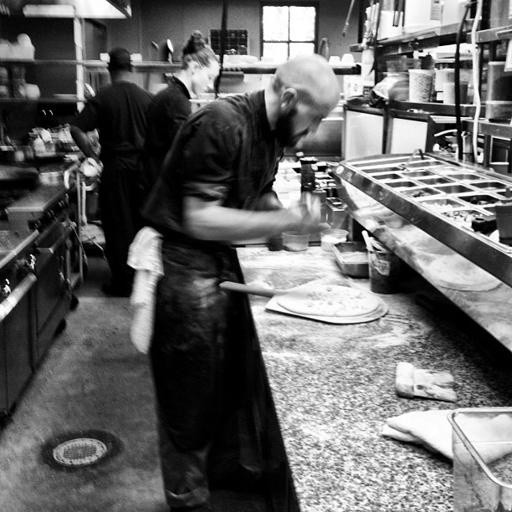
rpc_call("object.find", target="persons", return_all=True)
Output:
[125,52,342,511]
[128,28,221,243]
[68,46,155,297]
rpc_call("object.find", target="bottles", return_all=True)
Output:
[311,184,327,224]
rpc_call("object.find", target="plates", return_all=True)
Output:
[53,92,78,100]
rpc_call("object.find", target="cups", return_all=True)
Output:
[365,243,406,295]
[25,84,41,100]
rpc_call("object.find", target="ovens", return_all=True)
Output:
[32,220,71,359]
